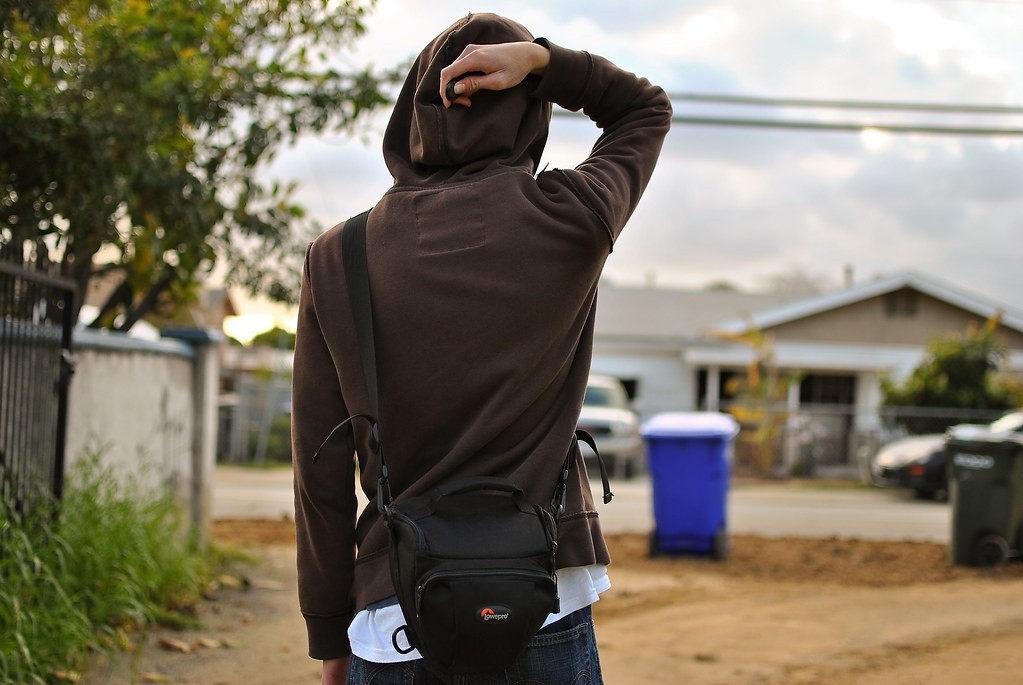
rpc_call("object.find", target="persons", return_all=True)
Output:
[292,13,672,685]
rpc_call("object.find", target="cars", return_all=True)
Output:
[870,412,1023,501]
[574,371,640,478]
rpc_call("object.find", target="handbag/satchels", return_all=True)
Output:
[382,477,559,674]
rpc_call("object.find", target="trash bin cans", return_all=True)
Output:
[941,424,1023,567]
[638,411,741,562]
